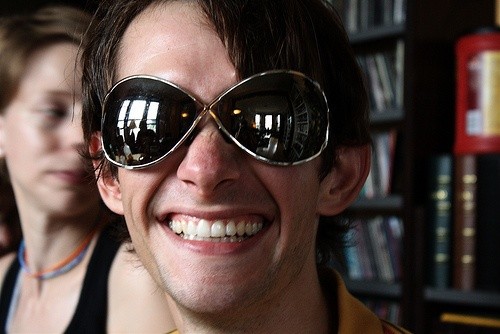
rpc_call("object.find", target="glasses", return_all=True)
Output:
[99,68,332,170]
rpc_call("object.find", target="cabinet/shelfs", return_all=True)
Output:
[326,0,496,332]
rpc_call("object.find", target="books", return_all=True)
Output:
[330,0,500,332]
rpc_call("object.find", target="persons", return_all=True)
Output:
[63,1,417,334]
[1,1,180,334]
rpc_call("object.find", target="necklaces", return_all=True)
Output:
[16,239,88,280]
[24,230,98,273]
